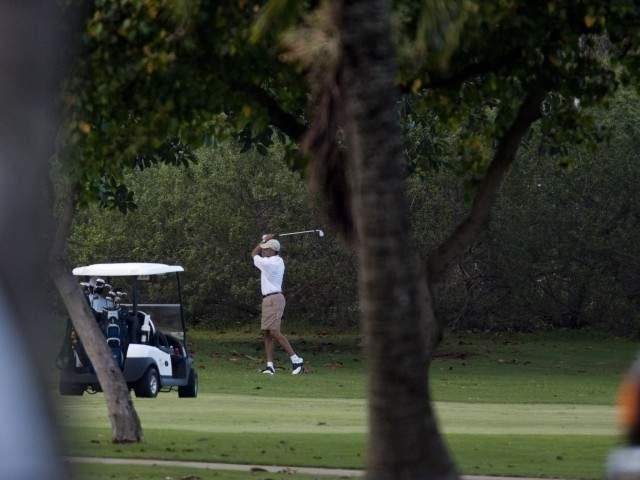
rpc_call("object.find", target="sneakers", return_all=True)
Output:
[291,357,304,375]
[260,367,275,374]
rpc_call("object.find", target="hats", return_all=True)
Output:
[261,239,281,251]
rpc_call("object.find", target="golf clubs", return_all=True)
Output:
[79,278,127,310]
[273,229,324,238]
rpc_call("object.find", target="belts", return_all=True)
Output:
[263,292,279,298]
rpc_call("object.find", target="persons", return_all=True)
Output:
[250,233,305,375]
